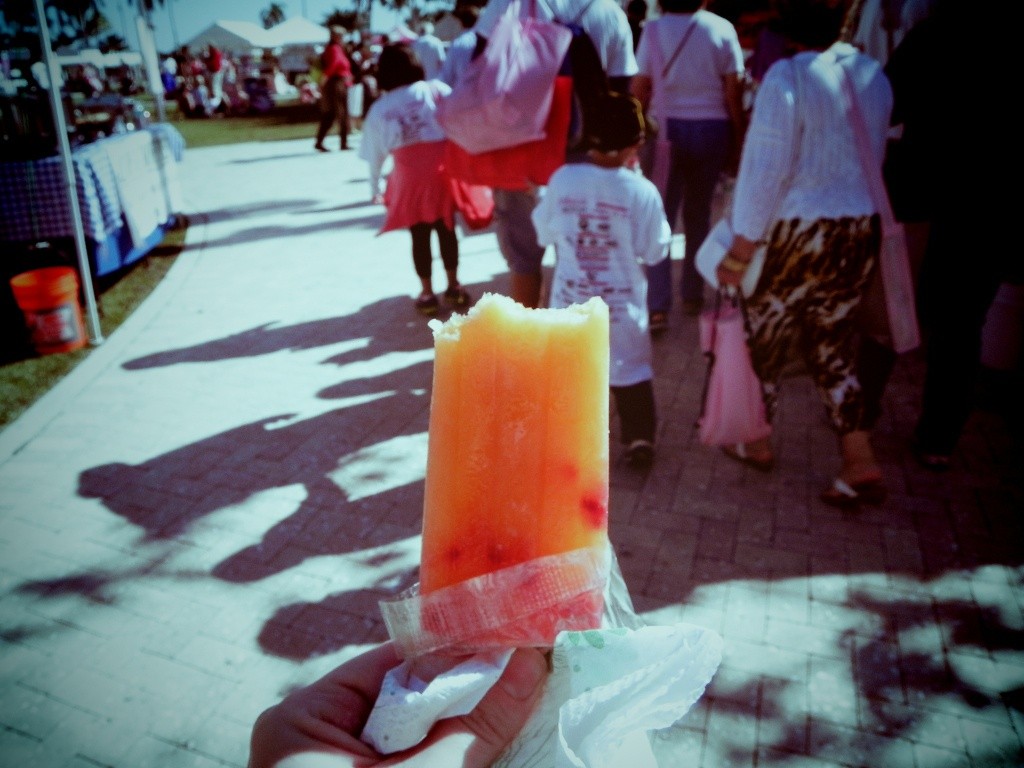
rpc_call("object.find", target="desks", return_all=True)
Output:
[0,122,182,280]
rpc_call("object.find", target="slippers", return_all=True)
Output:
[721,443,774,469]
[823,477,888,509]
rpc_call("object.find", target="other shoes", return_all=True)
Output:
[623,444,653,470]
[315,144,329,152]
[648,313,668,331]
[444,285,472,306]
[415,293,440,314]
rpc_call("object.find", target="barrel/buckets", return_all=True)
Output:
[11,266,88,357]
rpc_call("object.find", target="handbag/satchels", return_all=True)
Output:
[437,76,571,189]
[449,175,494,231]
[694,219,768,297]
[433,0,572,154]
[649,138,672,197]
[693,282,774,446]
[879,224,918,351]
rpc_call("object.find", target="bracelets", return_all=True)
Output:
[723,254,751,272]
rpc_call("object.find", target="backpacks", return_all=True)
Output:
[546,0,613,156]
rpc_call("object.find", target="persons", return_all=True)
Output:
[626,0,745,333]
[857,0,1024,476]
[414,5,480,86]
[627,0,646,53]
[248,639,551,768]
[529,90,672,474]
[719,0,894,508]
[314,26,389,151]
[356,41,473,314]
[468,0,638,307]
[159,41,321,119]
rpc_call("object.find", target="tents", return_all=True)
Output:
[54,51,143,71]
[182,18,330,53]
